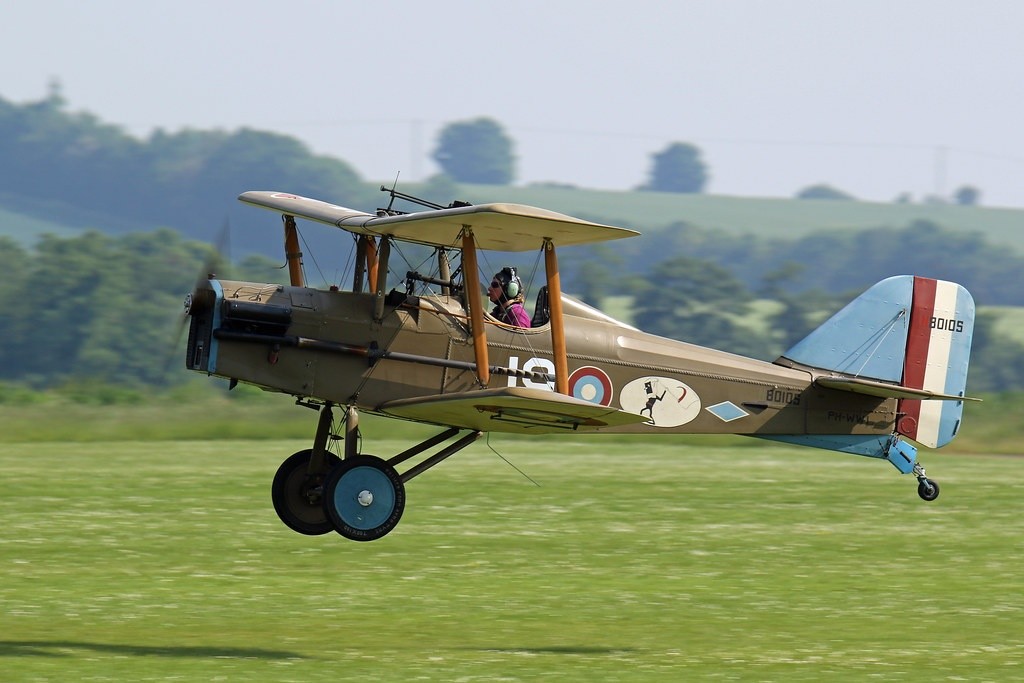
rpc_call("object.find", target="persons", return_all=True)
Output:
[489,268,530,330]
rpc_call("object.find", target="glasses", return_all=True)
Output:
[491,282,503,288]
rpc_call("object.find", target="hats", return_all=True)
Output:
[492,268,523,305]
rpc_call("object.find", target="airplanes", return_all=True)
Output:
[157,167,985,541]
